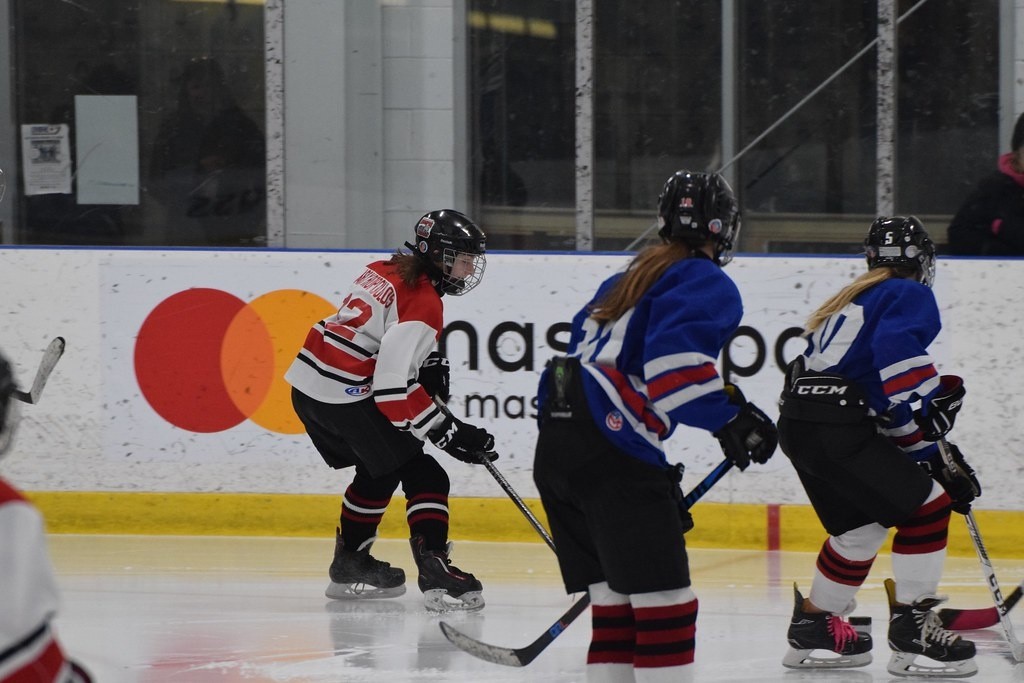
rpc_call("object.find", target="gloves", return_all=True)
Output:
[909,375,966,442]
[712,382,779,470]
[917,441,981,515]
[429,414,499,464]
[418,352,450,405]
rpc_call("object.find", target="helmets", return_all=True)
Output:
[864,214,936,288]
[414,210,486,297]
[657,168,744,268]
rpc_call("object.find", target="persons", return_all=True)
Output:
[24,50,146,244]
[147,58,266,246]
[778,215,981,662]
[0,356,92,683]
[947,112,1024,256]
[532,172,777,683]
[283,210,498,599]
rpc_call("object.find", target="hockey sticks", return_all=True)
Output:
[934,432,1023,663]
[10,335,67,404]
[477,451,559,555]
[439,432,765,669]
[937,586,1022,632]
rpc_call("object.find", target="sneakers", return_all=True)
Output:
[325,527,406,600]
[409,536,486,615]
[782,583,873,669]
[884,578,979,678]
